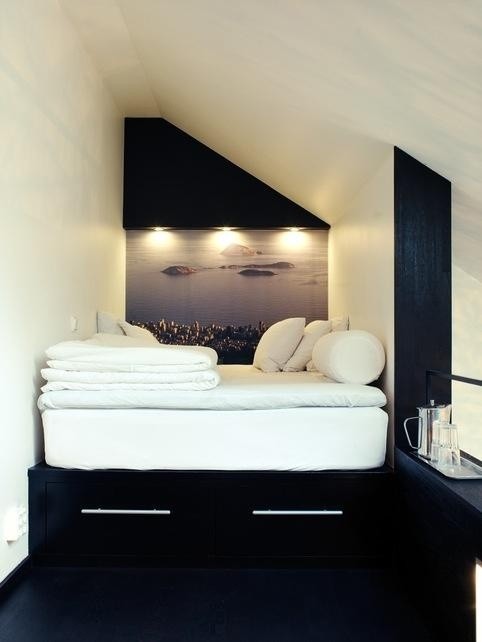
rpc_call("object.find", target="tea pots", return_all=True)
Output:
[402,398,453,459]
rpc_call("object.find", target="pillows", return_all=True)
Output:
[96,310,125,335]
[118,320,159,343]
[253,317,386,383]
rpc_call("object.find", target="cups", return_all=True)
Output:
[430,420,450,463]
[438,424,460,470]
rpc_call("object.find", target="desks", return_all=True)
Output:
[389,443,481,561]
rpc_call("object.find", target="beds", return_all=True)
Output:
[23,362,389,571]
